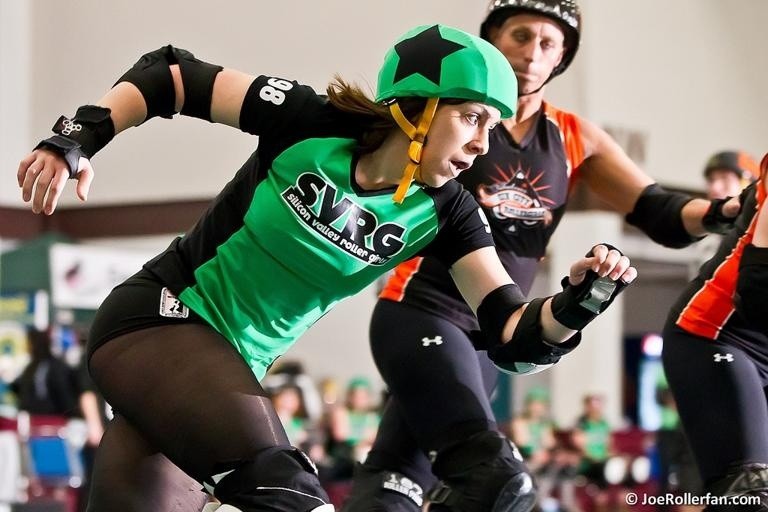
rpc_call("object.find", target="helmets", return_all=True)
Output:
[374,24,519,119]
[704,151,762,183]
[480,0,581,79]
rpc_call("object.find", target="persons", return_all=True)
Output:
[682,150,759,280]
[0,325,715,512]
[16,20,638,512]
[341,0,751,511]
[661,153,767,512]
